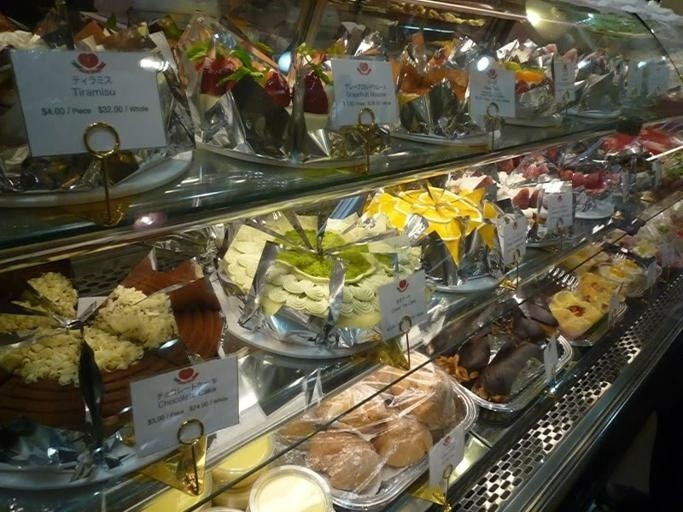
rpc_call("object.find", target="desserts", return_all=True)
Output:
[428,201,683,404]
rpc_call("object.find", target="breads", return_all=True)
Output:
[275,355,457,492]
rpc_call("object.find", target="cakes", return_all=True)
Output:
[0,251,222,469]
[215,198,425,348]
[1,15,194,194]
[363,122,683,288]
[172,15,671,162]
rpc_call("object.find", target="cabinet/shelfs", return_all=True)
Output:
[0,0,683,512]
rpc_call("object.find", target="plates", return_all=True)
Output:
[524,235,562,249]
[193,133,380,170]
[423,266,507,294]
[573,200,615,221]
[0,422,188,492]
[566,104,623,120]
[501,112,564,128]
[379,122,502,146]
[207,262,397,359]
[0,148,194,209]
